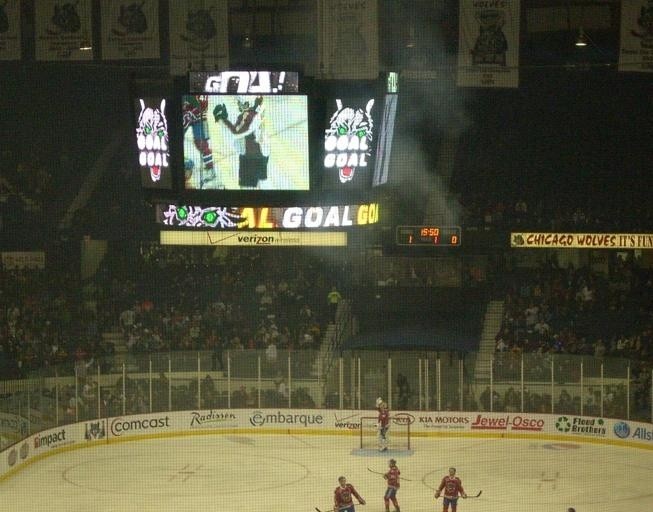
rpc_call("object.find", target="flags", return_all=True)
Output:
[0,0,22,63]
[169,0,230,79]
[33,0,96,60]
[318,1,379,78]
[458,1,522,89]
[100,0,163,59]
[616,1,653,75]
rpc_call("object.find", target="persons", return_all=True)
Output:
[2,254,345,378]
[382,457,401,512]
[332,474,365,512]
[374,398,390,453]
[3,147,146,251]
[485,250,652,363]
[212,93,273,191]
[351,366,652,430]
[39,375,347,419]
[178,92,216,188]
[435,467,467,511]
[380,189,652,234]
